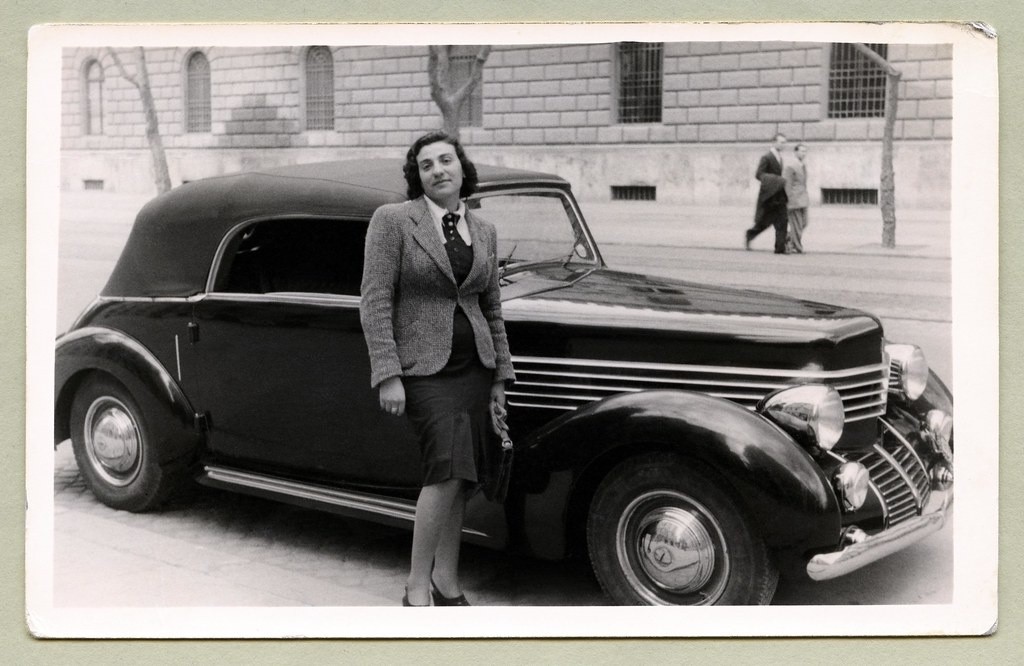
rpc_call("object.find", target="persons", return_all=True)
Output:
[361,131,515,606]
[746,134,787,253]
[782,144,809,254]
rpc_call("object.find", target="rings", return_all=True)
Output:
[391,408,397,411]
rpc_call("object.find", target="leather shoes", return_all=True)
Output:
[430,577,470,606]
[402,585,430,607]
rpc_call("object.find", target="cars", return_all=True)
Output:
[54,158,952,607]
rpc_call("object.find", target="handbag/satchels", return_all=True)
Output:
[482,400,514,504]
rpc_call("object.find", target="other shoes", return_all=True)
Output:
[774,249,791,254]
[746,230,751,250]
